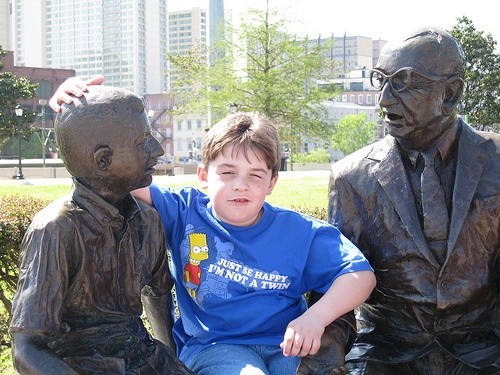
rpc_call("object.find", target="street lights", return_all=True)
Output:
[15,105,24,179]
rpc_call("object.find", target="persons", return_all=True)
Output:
[9,84,193,375]
[48,74,376,375]
[304,26,499,375]
